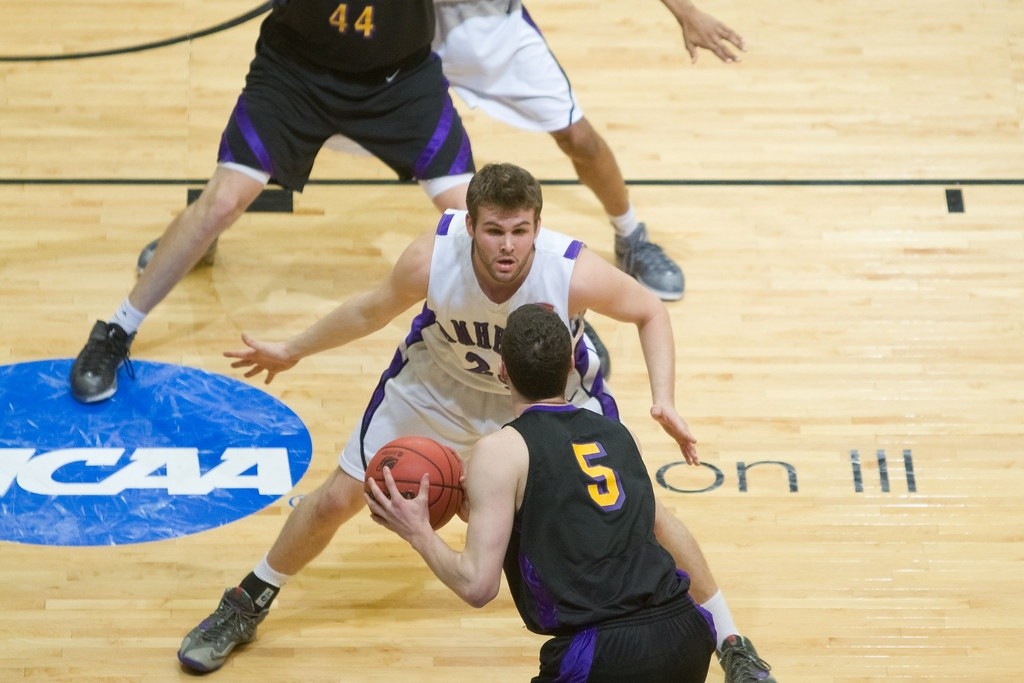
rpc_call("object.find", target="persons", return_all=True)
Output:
[69,0,610,406]
[361,304,718,683]
[177,164,774,683]
[136,0,744,300]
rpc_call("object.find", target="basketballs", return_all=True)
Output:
[363,434,466,533]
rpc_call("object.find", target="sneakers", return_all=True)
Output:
[615,222,685,301]
[135,225,219,278]
[69,320,137,404]
[714,636,778,683]
[176,586,270,674]
